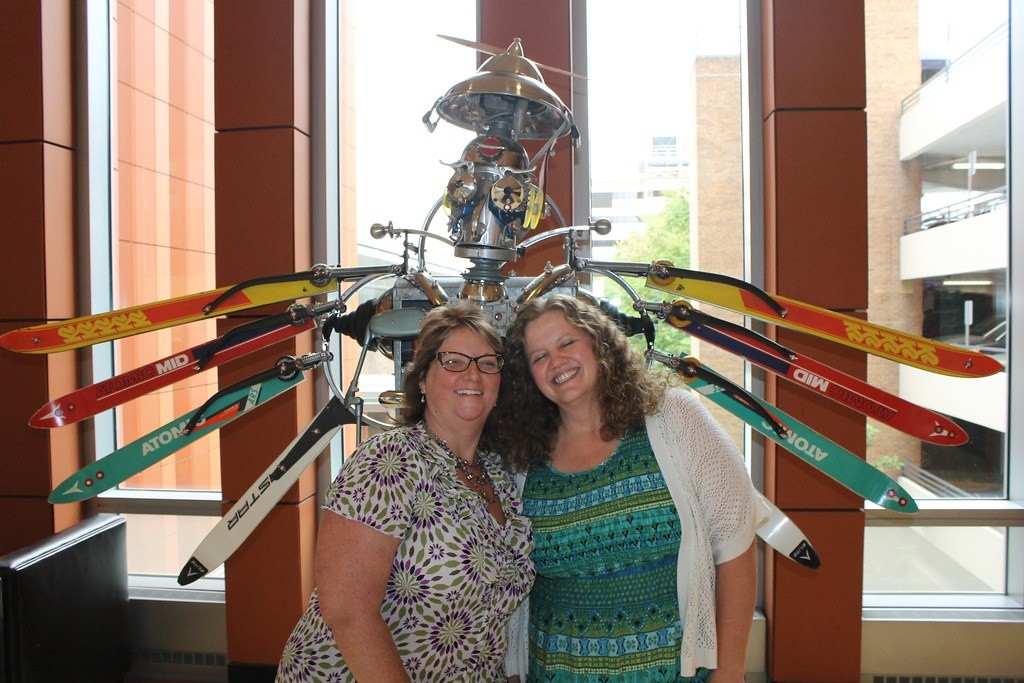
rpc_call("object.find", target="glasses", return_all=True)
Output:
[424,350,506,376]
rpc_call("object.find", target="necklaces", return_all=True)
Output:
[419,416,500,503]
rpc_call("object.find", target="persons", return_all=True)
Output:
[274,302,536,683]
[505,295,756,683]
